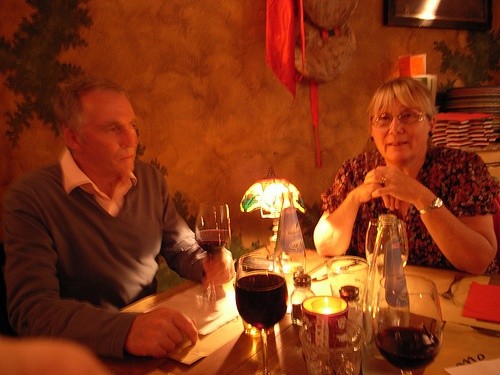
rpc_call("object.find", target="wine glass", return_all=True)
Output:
[235,253,288,375]
[195,202,231,314]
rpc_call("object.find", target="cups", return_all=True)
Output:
[299,318,362,375]
[326,257,370,344]
[375,274,445,375]
[366,218,408,278]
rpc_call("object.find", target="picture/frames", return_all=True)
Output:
[383,0,493,31]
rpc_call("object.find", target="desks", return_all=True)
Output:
[102,243,500,375]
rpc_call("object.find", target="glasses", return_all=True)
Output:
[370,111,431,126]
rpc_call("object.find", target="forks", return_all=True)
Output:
[439,274,466,300]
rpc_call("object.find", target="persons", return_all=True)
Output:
[313,77,500,276]
[0,75,236,360]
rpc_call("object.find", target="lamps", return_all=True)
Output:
[240,167,306,251]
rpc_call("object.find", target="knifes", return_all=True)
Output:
[311,262,359,282]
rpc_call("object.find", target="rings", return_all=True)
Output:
[379,175,387,186]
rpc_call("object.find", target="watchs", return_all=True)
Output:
[419,197,443,215]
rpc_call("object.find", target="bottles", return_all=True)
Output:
[339,285,364,351]
[273,192,307,314]
[361,214,410,361]
[290,275,316,326]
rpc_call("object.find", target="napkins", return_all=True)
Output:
[462,281,500,321]
[444,359,500,375]
